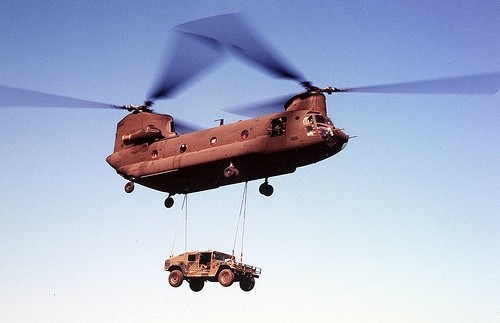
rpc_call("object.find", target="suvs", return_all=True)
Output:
[165,251,258,292]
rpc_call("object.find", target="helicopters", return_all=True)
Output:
[0,13,499,224]
